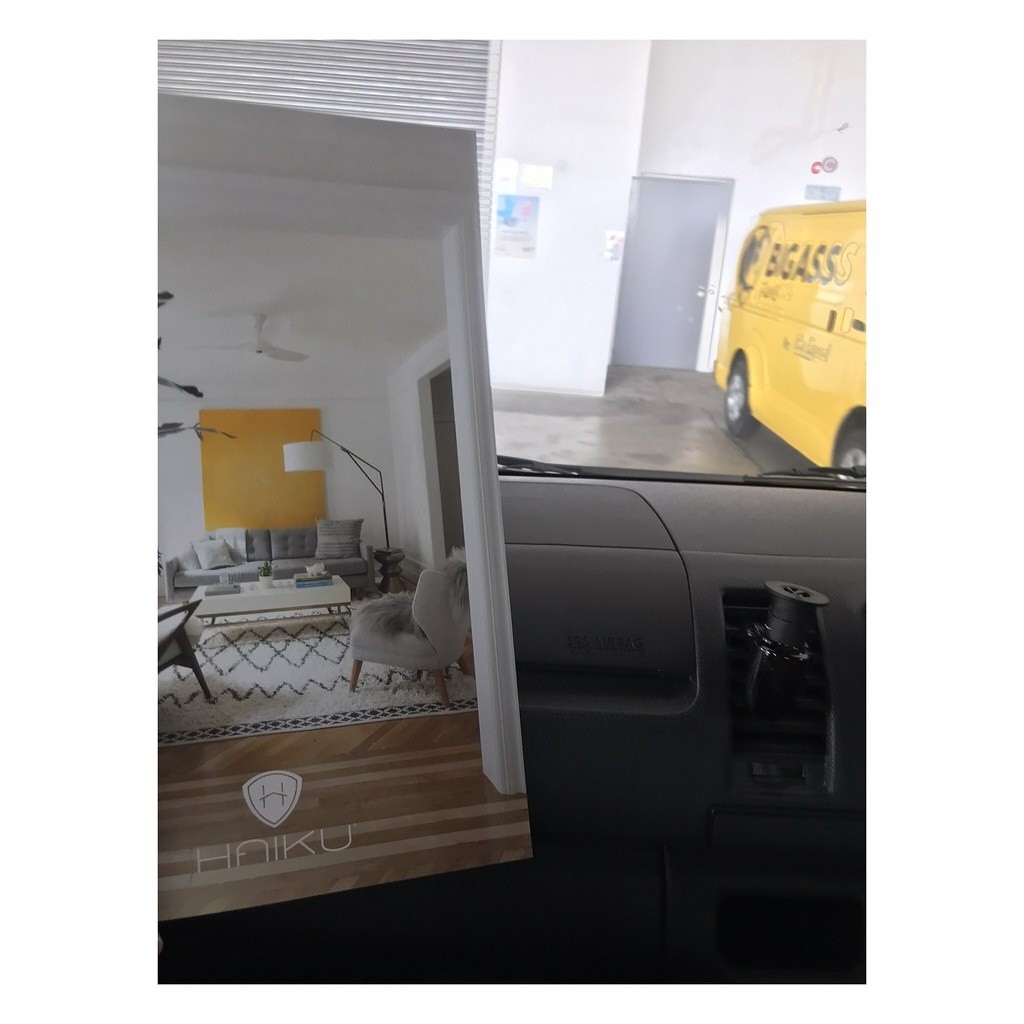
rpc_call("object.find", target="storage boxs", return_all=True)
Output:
[293,571,333,588]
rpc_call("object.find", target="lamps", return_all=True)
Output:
[283,429,390,548]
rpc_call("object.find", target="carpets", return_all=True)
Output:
[158,595,478,747]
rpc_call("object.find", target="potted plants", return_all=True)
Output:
[257,562,274,588]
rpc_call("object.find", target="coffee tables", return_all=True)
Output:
[189,575,351,629]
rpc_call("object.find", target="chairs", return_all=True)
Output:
[158,597,213,699]
[349,567,472,708]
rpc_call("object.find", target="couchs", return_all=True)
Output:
[165,525,375,604]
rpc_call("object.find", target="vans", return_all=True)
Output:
[714,198,867,484]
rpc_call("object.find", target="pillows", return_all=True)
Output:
[193,537,237,570]
[316,518,365,559]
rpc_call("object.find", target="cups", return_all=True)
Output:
[219,574,228,584]
